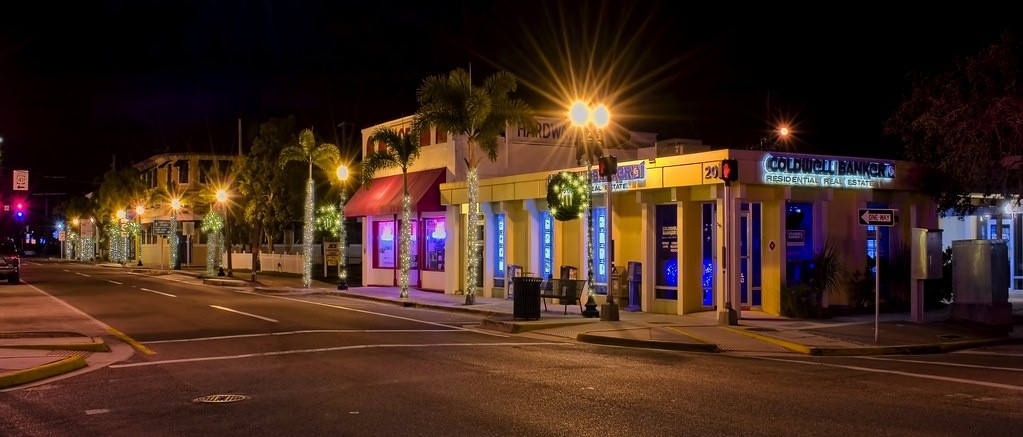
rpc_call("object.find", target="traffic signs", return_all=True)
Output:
[152,228,171,235]
[858,208,894,227]
[153,220,171,227]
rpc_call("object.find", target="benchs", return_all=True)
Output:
[540,279,587,315]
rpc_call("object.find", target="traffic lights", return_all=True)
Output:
[15,201,24,220]
[721,159,738,182]
[599,155,617,176]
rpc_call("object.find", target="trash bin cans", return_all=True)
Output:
[511,276,543,319]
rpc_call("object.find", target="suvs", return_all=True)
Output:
[0,239,22,285]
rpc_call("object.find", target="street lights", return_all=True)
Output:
[216,189,234,277]
[568,99,610,317]
[136,205,144,266]
[172,198,182,270]
[336,165,349,291]
